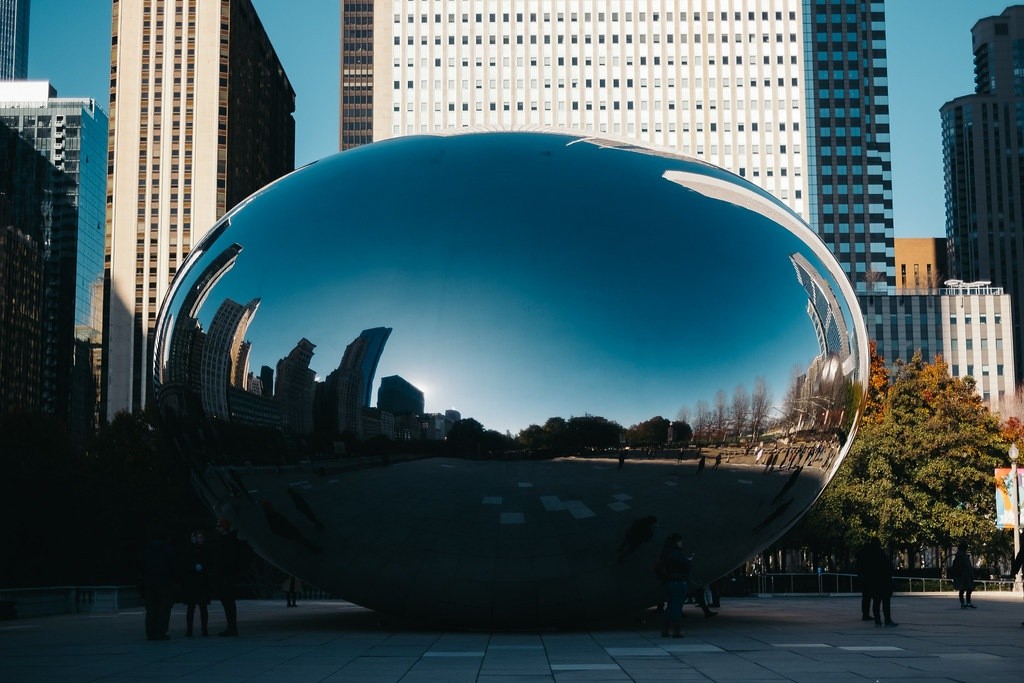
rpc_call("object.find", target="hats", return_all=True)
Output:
[221,517,231,530]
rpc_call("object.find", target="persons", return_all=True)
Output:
[954,543,977,608]
[162,396,841,609]
[178,530,212,637]
[853,536,899,628]
[281,574,302,607]
[657,536,735,637]
[215,517,241,636]
[138,529,180,640]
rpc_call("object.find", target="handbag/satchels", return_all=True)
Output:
[282,580,289,592]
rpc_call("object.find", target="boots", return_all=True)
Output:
[874,612,881,627]
[186,612,194,636]
[217,615,238,636]
[703,604,718,618]
[884,613,898,627]
[201,613,209,636]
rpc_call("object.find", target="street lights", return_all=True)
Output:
[1009,443,1023,593]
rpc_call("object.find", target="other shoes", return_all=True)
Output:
[673,633,684,637]
[684,599,720,607]
[148,635,170,640]
[961,603,977,609]
[661,632,670,636]
[861,616,872,621]
[287,604,297,607]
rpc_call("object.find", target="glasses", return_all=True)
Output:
[195,535,203,539]
[216,524,222,527]
